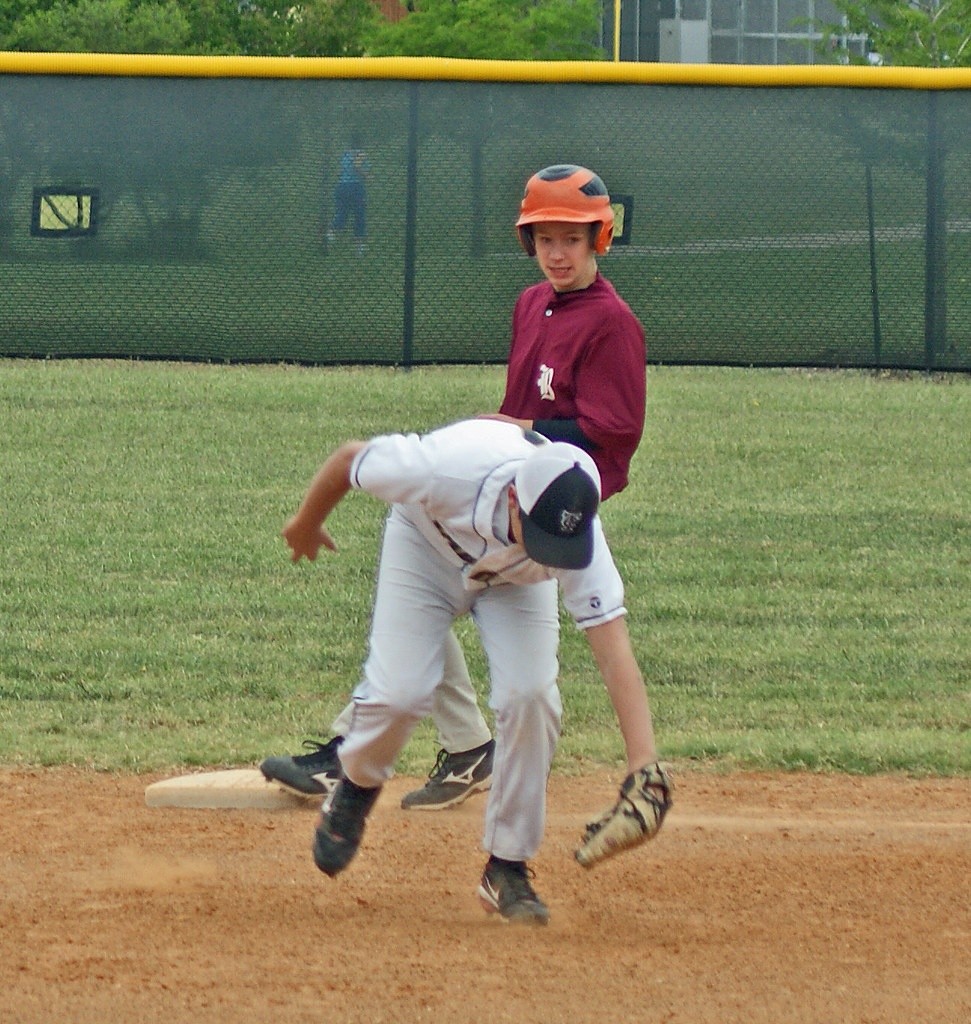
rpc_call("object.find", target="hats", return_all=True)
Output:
[515,441,601,571]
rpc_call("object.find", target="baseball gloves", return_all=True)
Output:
[575,761,674,872]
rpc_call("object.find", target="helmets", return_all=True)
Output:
[516,164,615,257]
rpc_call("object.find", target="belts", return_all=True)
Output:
[433,523,479,574]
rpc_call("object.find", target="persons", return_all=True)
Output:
[282,419,670,925]
[327,134,371,253]
[259,165,646,811]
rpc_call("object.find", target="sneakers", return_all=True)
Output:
[401,738,496,814]
[258,735,343,804]
[478,857,559,935]
[312,775,383,881]
